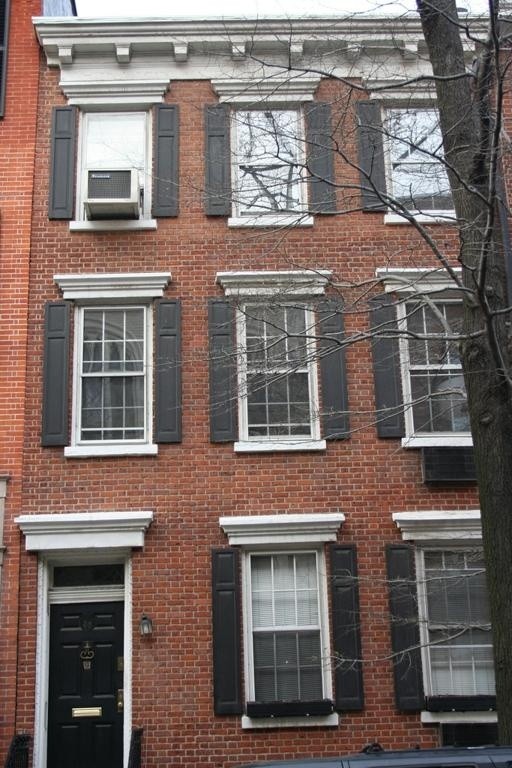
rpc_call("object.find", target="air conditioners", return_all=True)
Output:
[81,166,139,213]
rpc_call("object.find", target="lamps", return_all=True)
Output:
[138,613,153,635]
